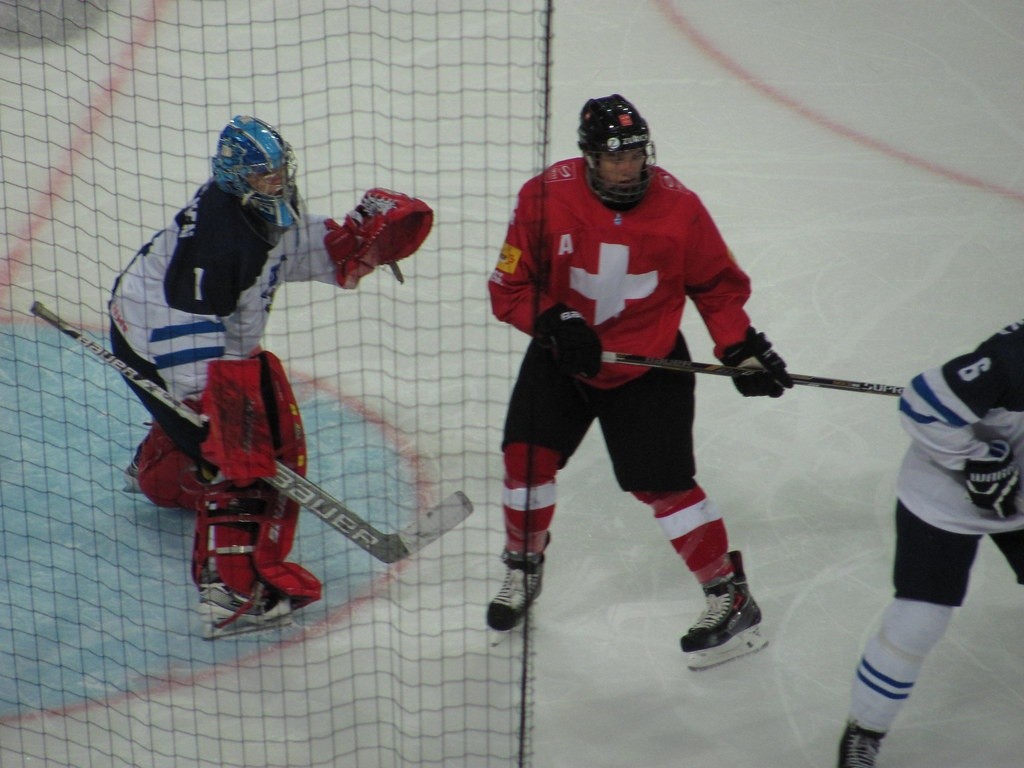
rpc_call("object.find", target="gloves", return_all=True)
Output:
[713,325,793,399]
[532,301,603,380]
[964,439,1021,519]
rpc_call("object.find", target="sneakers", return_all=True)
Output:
[486,530,551,647]
[680,550,769,672]
[836,721,887,768]
[199,569,294,640]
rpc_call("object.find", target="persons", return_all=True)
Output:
[837,321,1024,767]
[109,114,408,641]
[486,93,795,653]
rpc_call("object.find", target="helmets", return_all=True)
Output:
[577,93,657,213]
[212,114,298,227]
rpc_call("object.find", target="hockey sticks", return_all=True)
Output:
[601,351,903,401]
[28,301,474,564]
